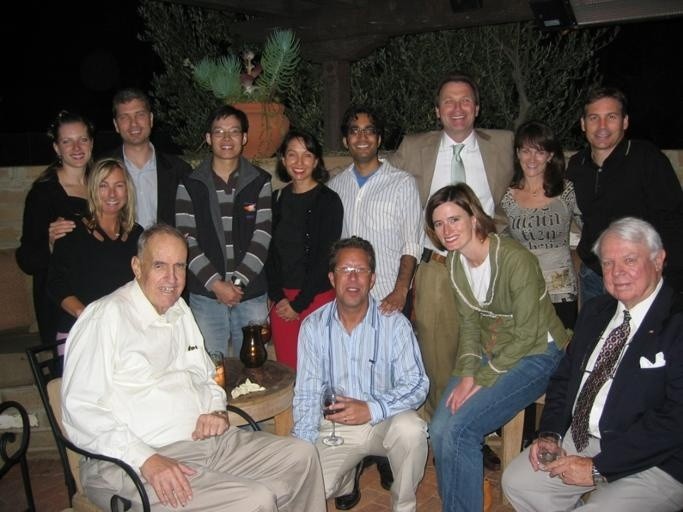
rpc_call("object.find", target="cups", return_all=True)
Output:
[209,351,226,389]
[538,432,562,472]
[241,324,272,368]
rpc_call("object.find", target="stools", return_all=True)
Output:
[484,391,548,506]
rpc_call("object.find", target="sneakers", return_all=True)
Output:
[477,440,502,475]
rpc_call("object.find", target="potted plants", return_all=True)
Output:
[183,23,300,163]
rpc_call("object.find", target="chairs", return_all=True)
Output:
[28,339,151,511]
[0,401,36,512]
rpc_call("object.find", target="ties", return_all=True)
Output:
[568,308,635,453]
[449,144,466,186]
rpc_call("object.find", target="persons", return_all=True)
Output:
[502,217,683,512]
[326,106,425,488]
[47,157,145,370]
[294,236,430,512]
[501,120,585,449]
[104,85,193,229]
[566,89,683,306]
[60,223,327,511]
[426,184,569,512]
[16,110,96,349]
[173,106,272,362]
[265,128,343,368]
[389,73,515,470]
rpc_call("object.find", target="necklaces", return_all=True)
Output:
[471,236,484,268]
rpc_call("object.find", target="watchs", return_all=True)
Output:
[211,410,227,417]
[231,276,245,289]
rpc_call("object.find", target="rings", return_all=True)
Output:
[561,472,565,478]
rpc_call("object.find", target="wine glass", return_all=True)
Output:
[319,385,345,447]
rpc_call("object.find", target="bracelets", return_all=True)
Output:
[592,464,605,487]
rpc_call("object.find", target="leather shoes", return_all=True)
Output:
[375,454,394,489]
[334,457,364,510]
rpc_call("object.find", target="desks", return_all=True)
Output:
[213,358,296,436]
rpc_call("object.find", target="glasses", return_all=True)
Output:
[348,127,376,137]
[211,128,241,137]
[338,266,371,277]
